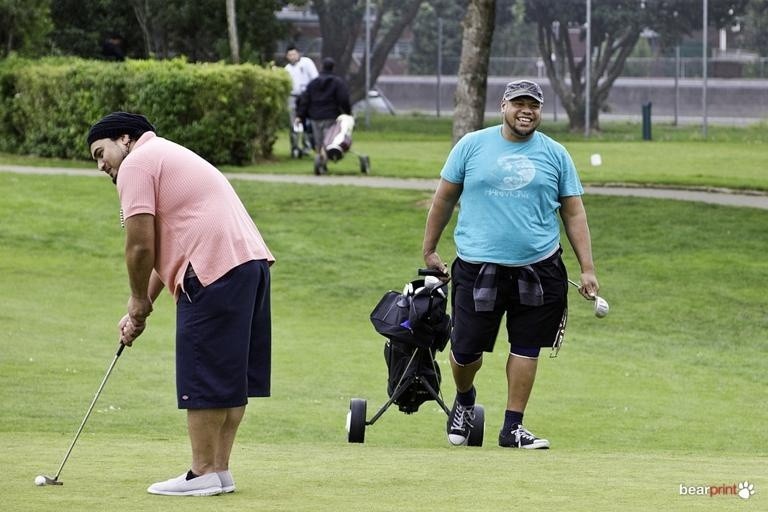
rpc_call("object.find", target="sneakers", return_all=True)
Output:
[215,471,235,494]
[146,470,222,496]
[446,388,476,447]
[499,425,550,449]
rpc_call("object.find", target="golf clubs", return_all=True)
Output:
[44,343,125,485]
[568,279,609,317]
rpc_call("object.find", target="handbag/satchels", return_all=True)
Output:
[368,288,451,347]
[380,341,441,413]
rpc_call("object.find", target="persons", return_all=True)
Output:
[283,43,318,158]
[422,80,600,451]
[295,56,353,174]
[88,111,276,497]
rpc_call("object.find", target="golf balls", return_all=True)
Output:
[35,476,46,485]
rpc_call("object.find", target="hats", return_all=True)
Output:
[321,57,334,67]
[87,110,155,147]
[501,79,543,103]
